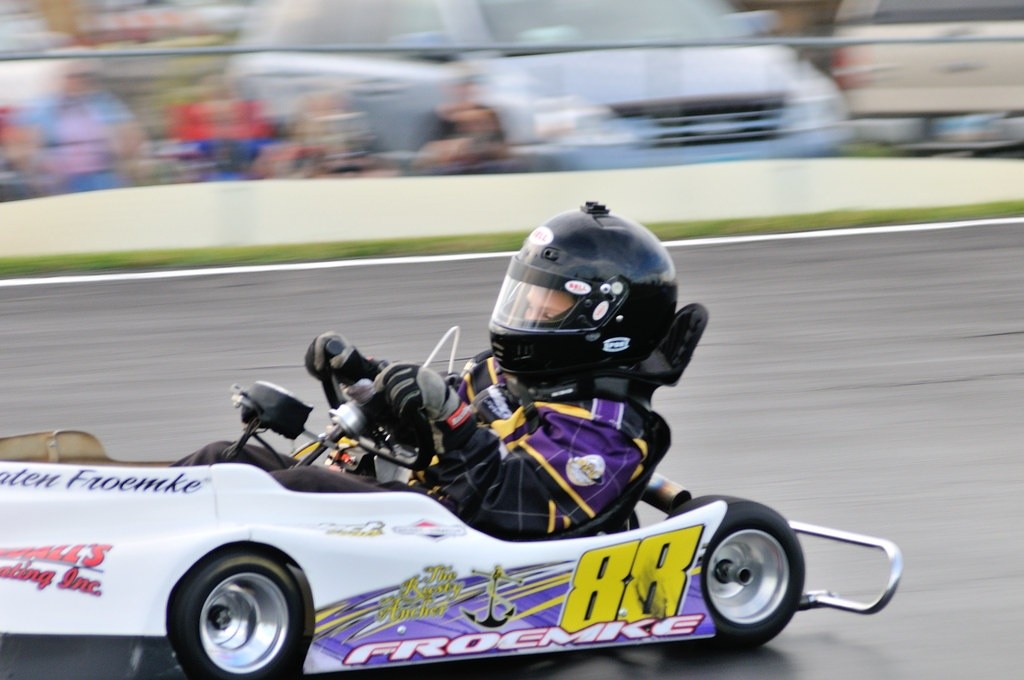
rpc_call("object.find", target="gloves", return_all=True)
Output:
[376,361,476,450]
[305,330,377,387]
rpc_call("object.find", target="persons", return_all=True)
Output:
[405,64,514,171]
[172,203,679,541]
[0,46,385,198]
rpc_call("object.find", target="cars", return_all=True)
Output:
[224,0,849,179]
[0,0,150,199]
[825,0,1024,160]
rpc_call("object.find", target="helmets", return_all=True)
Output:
[489,200,677,374]
